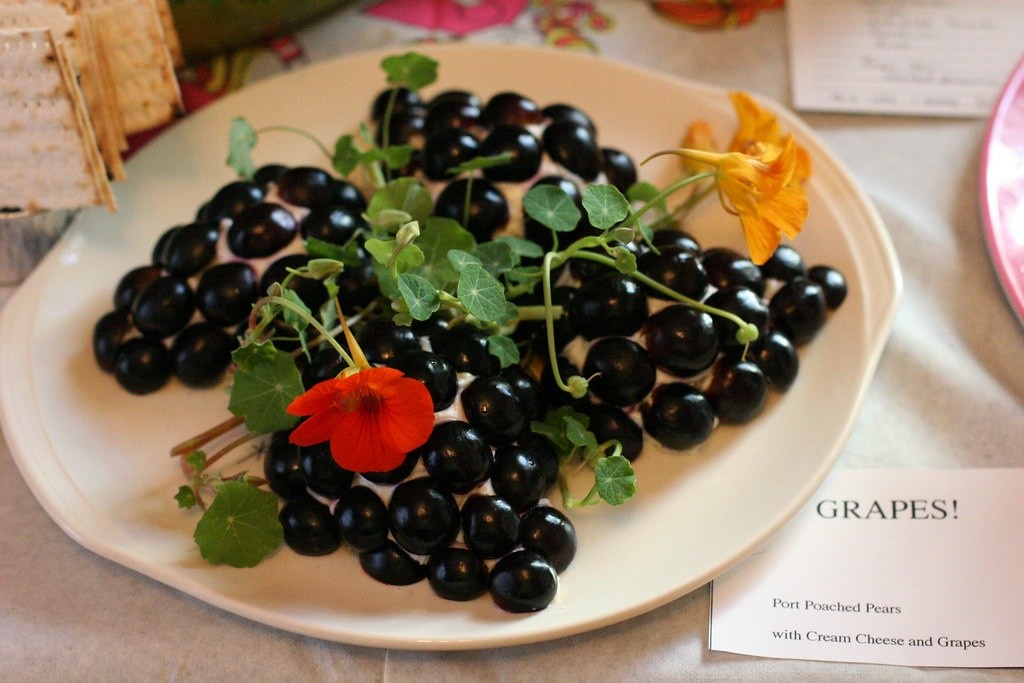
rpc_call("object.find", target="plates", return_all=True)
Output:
[977,55,1024,331]
[0,42,905,651]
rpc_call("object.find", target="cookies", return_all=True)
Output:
[0,0,184,214]
